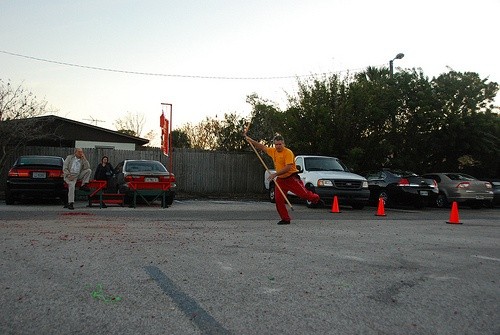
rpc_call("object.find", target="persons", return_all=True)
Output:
[245,134,326,225]
[94,156,114,180]
[62,148,92,210]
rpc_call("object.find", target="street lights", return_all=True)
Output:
[389,52,405,144]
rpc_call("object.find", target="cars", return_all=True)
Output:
[356,168,439,209]
[113,159,176,205]
[5,155,68,205]
[422,172,500,210]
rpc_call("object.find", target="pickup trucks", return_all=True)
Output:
[263,155,371,210]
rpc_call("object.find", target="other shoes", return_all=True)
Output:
[67,203,75,209]
[79,185,90,192]
[277,220,290,224]
[318,199,324,207]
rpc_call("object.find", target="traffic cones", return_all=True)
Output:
[330,196,341,213]
[445,201,464,224]
[374,197,387,216]
